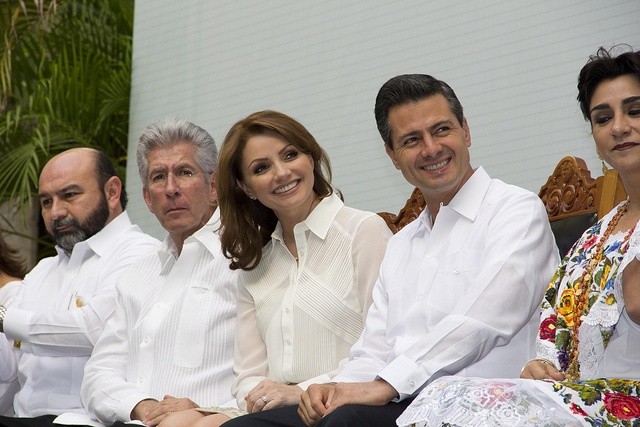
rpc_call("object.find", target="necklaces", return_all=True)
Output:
[573,199,639,358]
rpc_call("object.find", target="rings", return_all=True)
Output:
[261,394,267,403]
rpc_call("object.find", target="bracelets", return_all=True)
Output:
[0,306,8,333]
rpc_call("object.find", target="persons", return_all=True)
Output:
[396,43,640,427]
[0,147,162,427]
[220,74,561,427]
[156,110,393,427]
[80,116,237,427]
[0,235,30,418]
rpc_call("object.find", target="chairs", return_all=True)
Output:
[537,156,618,262]
